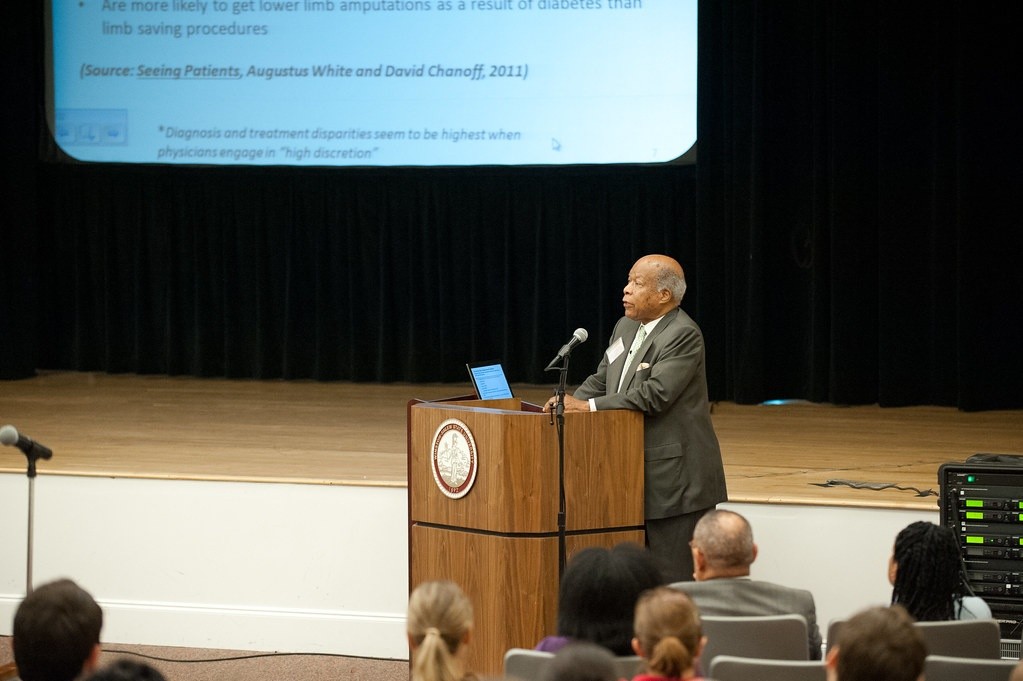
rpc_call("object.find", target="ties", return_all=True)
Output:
[620,326,646,389]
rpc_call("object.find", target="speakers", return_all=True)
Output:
[937,452,1023,623]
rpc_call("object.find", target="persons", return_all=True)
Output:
[826,606,930,681]
[544,644,627,681]
[13,578,166,681]
[407,582,480,681]
[536,543,663,658]
[837,521,991,623]
[631,587,707,681]
[668,512,822,661]
[543,255,728,583]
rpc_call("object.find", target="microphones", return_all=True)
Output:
[544,327,588,371]
[0,424,52,461]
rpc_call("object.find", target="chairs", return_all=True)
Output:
[697,612,808,676]
[911,619,1001,660]
[708,654,827,681]
[503,648,645,681]
[923,655,1013,681]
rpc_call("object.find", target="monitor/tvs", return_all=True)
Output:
[465,360,515,400]
